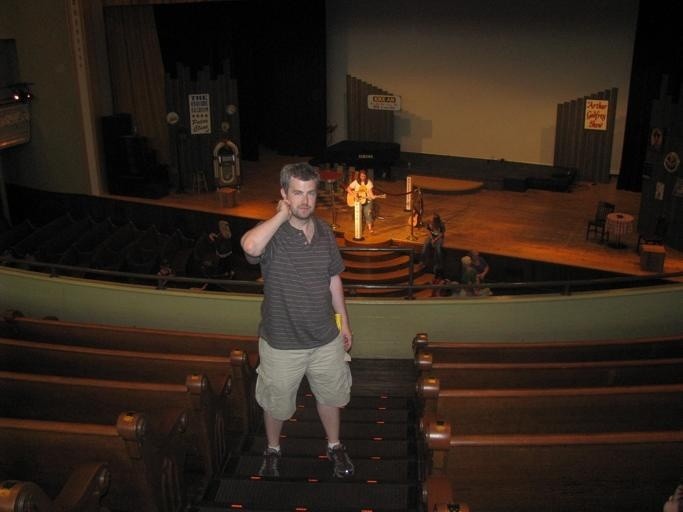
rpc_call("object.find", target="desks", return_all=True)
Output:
[607,211,635,249]
[639,243,666,274]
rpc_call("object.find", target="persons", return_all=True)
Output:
[345,169,374,233]
[240,163,355,478]
[209,221,236,279]
[430,250,493,297]
[418,214,445,275]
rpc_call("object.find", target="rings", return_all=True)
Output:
[346,334,351,339]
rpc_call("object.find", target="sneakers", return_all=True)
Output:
[257,445,284,481]
[323,442,358,481]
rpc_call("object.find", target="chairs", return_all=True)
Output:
[637,213,670,256]
[586,199,615,243]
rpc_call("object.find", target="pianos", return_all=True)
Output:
[306,141,401,182]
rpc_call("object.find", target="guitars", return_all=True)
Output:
[347,191,385,207]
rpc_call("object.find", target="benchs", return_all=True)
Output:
[0,211,206,287]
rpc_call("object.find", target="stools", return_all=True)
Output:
[192,168,209,195]
